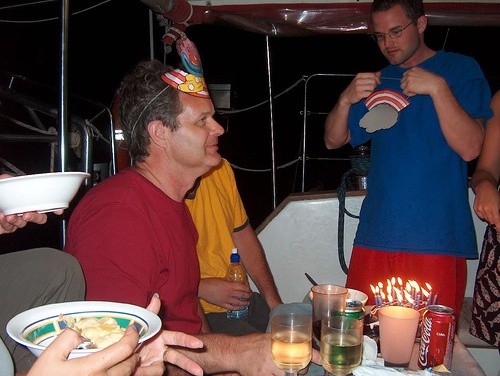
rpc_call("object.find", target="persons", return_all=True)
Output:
[0,174,205,376]
[66,34,325,376]
[326,0,494,320]
[468,93,500,348]
[184,158,283,337]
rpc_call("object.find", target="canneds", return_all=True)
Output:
[417,304,456,372]
[326,299,364,366]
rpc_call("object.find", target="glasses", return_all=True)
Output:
[369,17,416,40]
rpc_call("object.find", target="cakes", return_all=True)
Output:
[369,299,431,339]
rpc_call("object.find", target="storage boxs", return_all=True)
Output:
[207,84,231,110]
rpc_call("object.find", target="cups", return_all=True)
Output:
[312,284,348,341]
[378,306,420,368]
[320,316,364,376]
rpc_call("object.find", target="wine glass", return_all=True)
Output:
[272,313,312,376]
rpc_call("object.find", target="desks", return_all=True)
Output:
[266,302,487,376]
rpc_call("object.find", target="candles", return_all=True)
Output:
[369,277,438,310]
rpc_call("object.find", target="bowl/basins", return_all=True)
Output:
[6,301,162,367]
[308,288,369,307]
[0,172,91,216]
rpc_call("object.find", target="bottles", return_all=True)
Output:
[226,248,250,322]
